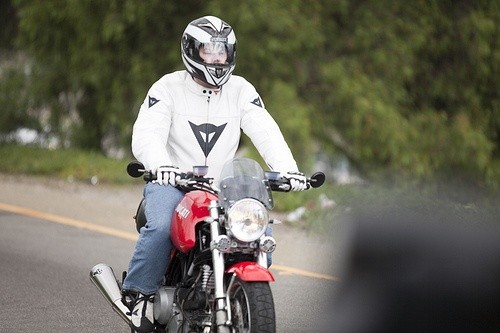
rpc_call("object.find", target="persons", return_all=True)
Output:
[121,15,307,333]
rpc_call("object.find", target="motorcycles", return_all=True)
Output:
[90,161,325,333]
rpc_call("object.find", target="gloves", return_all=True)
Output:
[157,166,183,187]
[280,171,308,193]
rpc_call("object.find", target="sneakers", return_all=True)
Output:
[132,293,156,333]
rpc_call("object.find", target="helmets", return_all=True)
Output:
[181,16,238,87]
